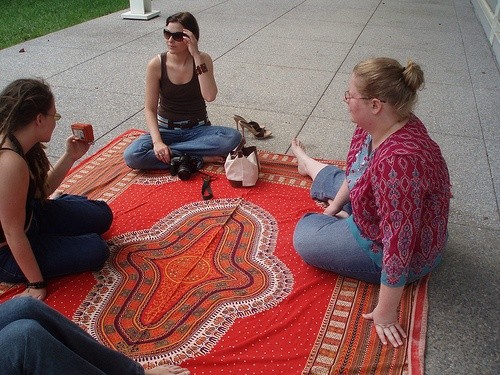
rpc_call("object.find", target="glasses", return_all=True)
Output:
[163,28,186,42]
[345,91,386,104]
[36,113,62,121]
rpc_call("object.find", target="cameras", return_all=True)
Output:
[170,153,202,180]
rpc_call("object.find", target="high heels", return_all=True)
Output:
[233,115,265,130]
[239,120,272,139]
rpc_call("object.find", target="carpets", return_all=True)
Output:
[0,127,428,375]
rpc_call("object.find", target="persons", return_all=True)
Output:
[291,57,451,348]
[0,294,190,375]
[0,79,114,300]
[124,11,245,181]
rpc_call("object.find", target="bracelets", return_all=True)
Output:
[195,63,208,76]
[27,280,48,290]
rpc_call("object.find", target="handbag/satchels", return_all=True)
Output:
[223,138,261,187]
